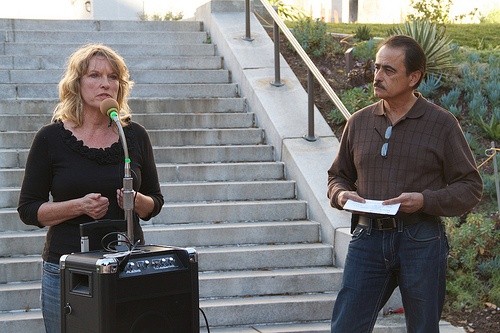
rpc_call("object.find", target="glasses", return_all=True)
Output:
[373,126,393,159]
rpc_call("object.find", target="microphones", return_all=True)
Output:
[100,97,119,122]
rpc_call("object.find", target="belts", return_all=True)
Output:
[357,215,425,231]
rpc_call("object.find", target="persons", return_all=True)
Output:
[328,36,482,333]
[18,43,164,333]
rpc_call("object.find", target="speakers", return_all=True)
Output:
[57,243,200,333]
